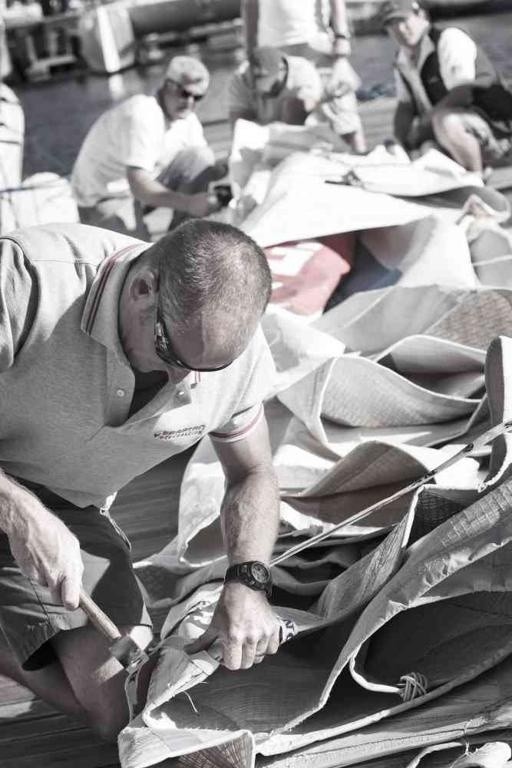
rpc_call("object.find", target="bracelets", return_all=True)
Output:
[334,32,352,41]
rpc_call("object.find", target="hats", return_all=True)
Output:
[167,56,209,96]
[380,0,413,24]
[250,47,280,94]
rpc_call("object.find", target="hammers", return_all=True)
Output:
[71,586,150,673]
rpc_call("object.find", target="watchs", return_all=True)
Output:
[222,560,275,601]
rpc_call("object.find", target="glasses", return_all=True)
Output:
[177,88,203,102]
[153,281,233,373]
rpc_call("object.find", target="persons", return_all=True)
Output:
[1,219,285,748]
[66,53,231,244]
[376,0,510,183]
[224,48,325,137]
[237,0,367,154]
